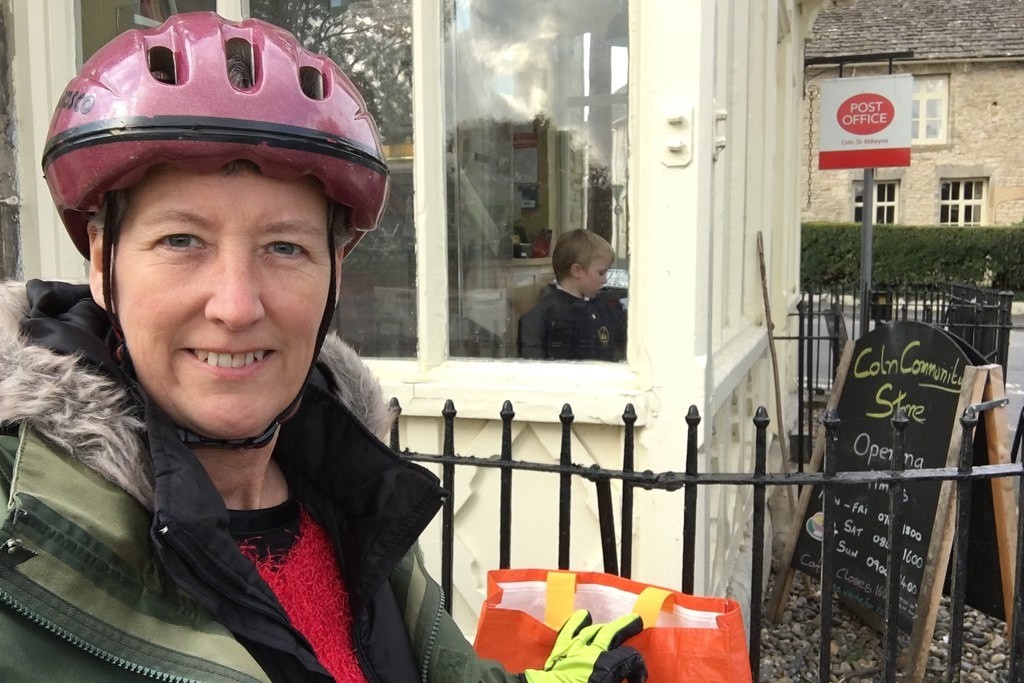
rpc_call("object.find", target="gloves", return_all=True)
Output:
[522,611,649,682]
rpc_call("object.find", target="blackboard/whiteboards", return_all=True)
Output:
[785,319,987,636]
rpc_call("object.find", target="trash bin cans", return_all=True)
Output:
[870,290,894,320]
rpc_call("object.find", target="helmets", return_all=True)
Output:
[40,8,391,260]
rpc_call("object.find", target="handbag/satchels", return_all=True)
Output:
[476,569,748,683]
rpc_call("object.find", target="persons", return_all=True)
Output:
[516,229,628,362]
[0,10,648,683]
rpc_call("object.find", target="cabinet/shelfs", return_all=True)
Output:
[464,256,558,358]
